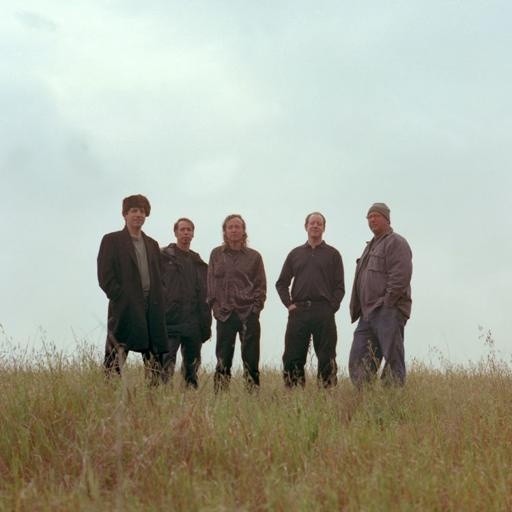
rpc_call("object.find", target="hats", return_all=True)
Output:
[368,203,390,224]
[122,194,151,217]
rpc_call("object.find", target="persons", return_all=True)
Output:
[203,212,268,398]
[348,201,414,396]
[274,211,346,394]
[157,216,213,391]
[96,194,172,389]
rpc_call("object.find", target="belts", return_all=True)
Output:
[294,300,329,306]
